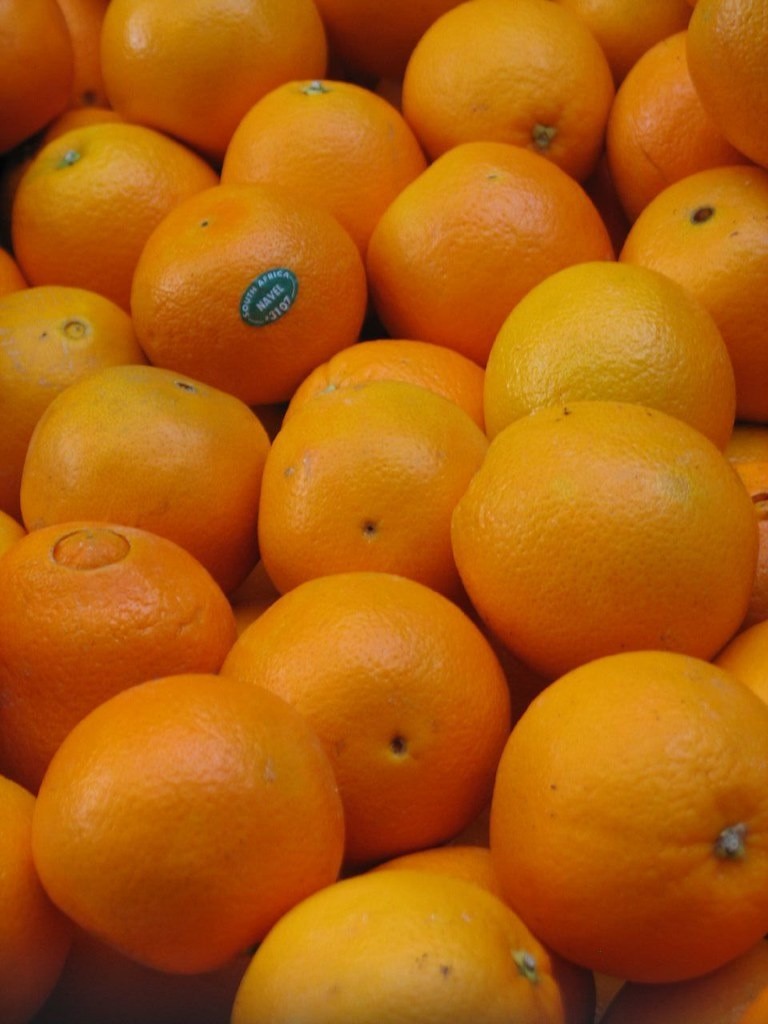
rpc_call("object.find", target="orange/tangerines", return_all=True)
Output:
[0,0,768,1024]
